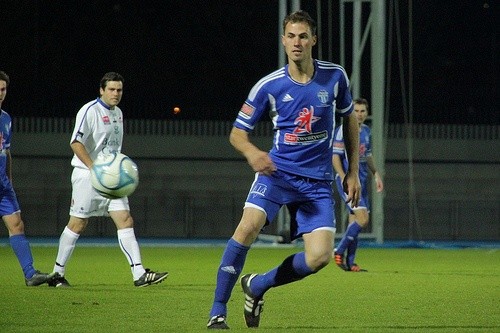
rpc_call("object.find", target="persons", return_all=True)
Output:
[331,98,384,271]
[206,10,362,330]
[49,73,169,288]
[0,71,61,287]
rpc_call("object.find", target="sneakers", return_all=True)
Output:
[240,273,265,328]
[333,248,346,271]
[207,315,230,329]
[133,268,169,287]
[48,274,71,287]
[25,270,59,286]
[349,264,368,272]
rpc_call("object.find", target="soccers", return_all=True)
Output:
[90,153,139,200]
